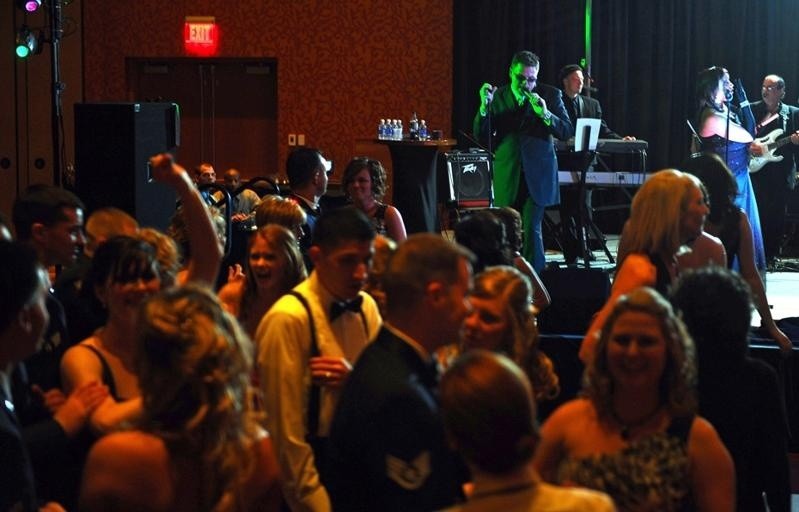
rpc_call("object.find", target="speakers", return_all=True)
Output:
[538,267,611,323]
[446,153,494,202]
[80,102,181,231]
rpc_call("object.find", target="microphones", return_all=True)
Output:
[520,87,535,100]
[723,87,730,101]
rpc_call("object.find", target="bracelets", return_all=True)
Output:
[544,110,551,121]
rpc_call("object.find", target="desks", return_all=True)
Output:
[370,135,457,235]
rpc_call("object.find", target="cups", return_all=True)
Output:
[432,130,443,140]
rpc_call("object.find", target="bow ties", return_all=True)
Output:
[328,294,362,323]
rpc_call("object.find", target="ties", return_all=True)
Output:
[573,97,581,118]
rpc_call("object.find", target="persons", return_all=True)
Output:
[555,62,638,263]
[742,72,798,260]
[686,64,770,308]
[470,50,572,273]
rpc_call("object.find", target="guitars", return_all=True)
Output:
[748,128,798,173]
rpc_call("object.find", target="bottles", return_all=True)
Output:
[378,117,428,142]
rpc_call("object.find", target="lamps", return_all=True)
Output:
[8,0,51,60]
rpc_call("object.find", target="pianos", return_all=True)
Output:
[552,139,648,154]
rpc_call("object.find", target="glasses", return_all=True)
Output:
[762,86,775,91]
[516,74,536,82]
